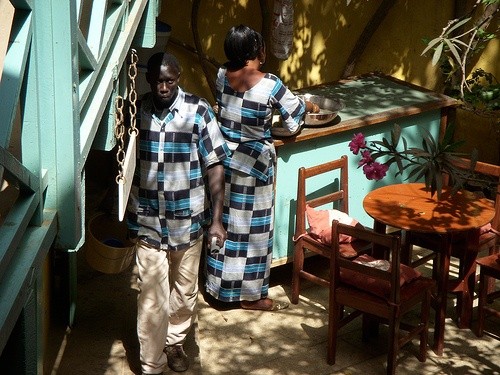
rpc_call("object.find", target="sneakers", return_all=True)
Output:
[164,345,189,372]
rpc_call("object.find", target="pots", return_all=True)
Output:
[271,108,305,136]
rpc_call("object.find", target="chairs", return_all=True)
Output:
[442,155,500,337]
[326,219,435,375]
[291,155,372,305]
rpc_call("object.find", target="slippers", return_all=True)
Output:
[265,299,290,311]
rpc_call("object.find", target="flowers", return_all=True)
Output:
[349,132,388,181]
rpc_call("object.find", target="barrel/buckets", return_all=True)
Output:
[85,210,137,274]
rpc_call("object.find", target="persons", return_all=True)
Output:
[121,52,232,375]
[201,21,321,312]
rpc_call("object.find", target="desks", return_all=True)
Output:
[363,183,496,355]
[270,72,456,269]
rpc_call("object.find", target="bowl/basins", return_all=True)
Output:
[296,94,344,125]
